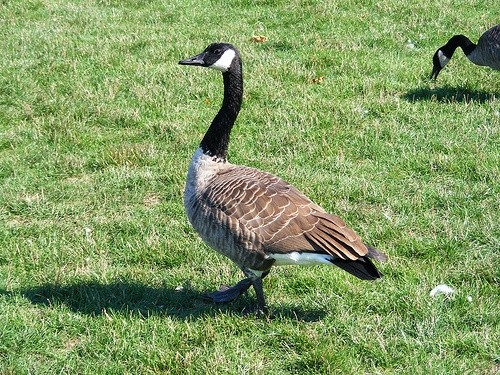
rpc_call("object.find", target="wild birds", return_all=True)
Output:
[429,23,500,82]
[177,42,389,318]
